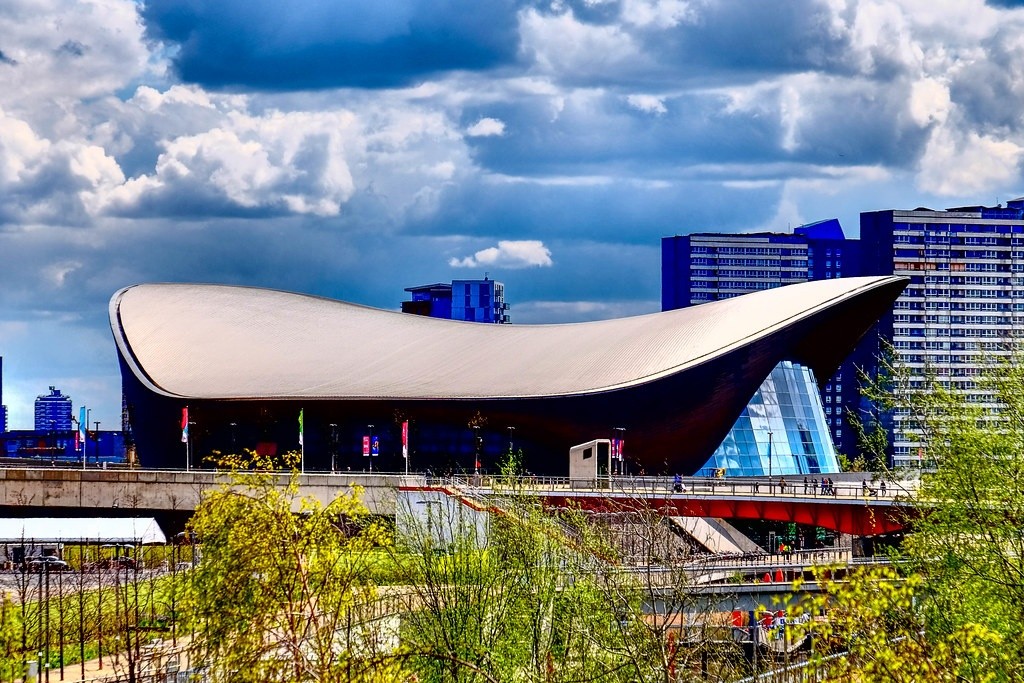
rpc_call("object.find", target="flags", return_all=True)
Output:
[180,408,188,442]
[402,422,408,458]
[475,453,481,468]
[362,436,379,456]
[78,407,85,442]
[732,610,743,627]
[611,428,626,462]
[298,410,303,445]
[763,610,785,639]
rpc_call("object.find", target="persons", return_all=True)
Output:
[780,542,785,555]
[826,478,835,496]
[783,543,791,553]
[820,476,828,495]
[789,541,797,554]
[674,473,682,493]
[812,479,818,495]
[863,479,867,496]
[778,477,787,494]
[804,476,809,494]
[755,482,759,493]
[880,480,886,497]
